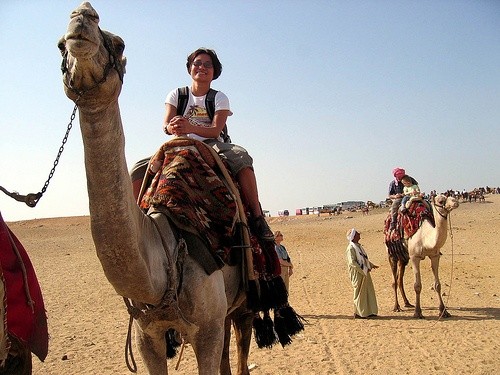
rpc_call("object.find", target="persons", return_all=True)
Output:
[344,228,380,320]
[162,46,277,242]
[273,230,294,300]
[388,167,419,230]
[396,175,424,213]
[421,185,500,204]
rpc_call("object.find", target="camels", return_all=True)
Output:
[56,1,275,375]
[0,214,32,375]
[385,191,460,318]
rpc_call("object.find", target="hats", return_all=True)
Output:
[393,168,405,177]
[346,228,356,240]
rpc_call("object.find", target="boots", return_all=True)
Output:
[390,214,397,230]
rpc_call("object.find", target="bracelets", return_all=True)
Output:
[163,124,173,136]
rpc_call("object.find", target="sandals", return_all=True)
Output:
[249,214,275,243]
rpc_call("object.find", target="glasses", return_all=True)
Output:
[192,61,214,67]
[280,234,283,236]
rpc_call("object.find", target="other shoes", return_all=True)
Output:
[401,208,406,212]
[368,314,377,317]
[355,314,367,318]
